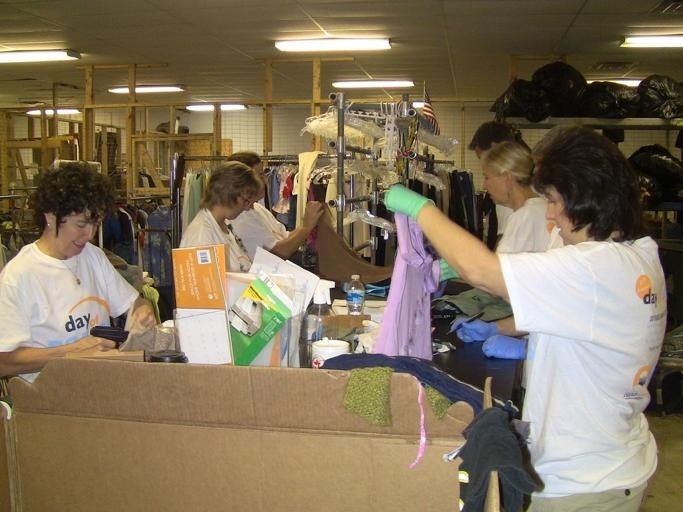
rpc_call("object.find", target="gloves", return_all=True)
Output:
[439,257,460,281]
[382,184,438,222]
[481,333,528,362]
[450,317,498,344]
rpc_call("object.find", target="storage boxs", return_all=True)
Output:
[172,244,321,368]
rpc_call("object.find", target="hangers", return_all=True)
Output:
[328,192,394,235]
[259,153,359,186]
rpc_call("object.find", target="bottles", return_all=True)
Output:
[346,274,365,315]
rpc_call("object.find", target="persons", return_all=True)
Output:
[177,160,253,272]
[0,162,157,383]
[224,152,325,264]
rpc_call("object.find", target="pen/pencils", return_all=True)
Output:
[446,311,486,336]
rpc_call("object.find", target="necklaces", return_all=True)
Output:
[59,254,81,285]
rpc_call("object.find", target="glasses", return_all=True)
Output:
[241,196,255,208]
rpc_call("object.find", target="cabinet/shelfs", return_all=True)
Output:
[492,116,683,252]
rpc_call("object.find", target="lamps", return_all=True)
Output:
[619,35,682,51]
[1,49,82,63]
[274,39,393,53]
[332,80,416,89]
[109,85,185,96]
[185,103,248,112]
